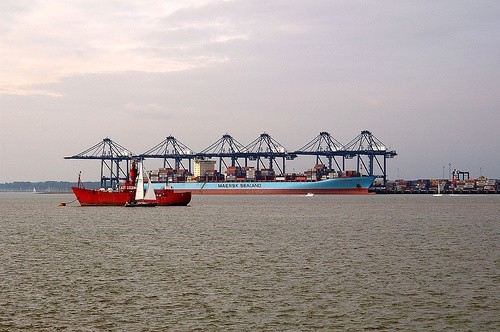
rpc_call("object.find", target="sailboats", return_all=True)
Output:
[124,157,156,208]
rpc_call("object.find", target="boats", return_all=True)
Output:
[71,158,192,205]
[120,170,373,196]
